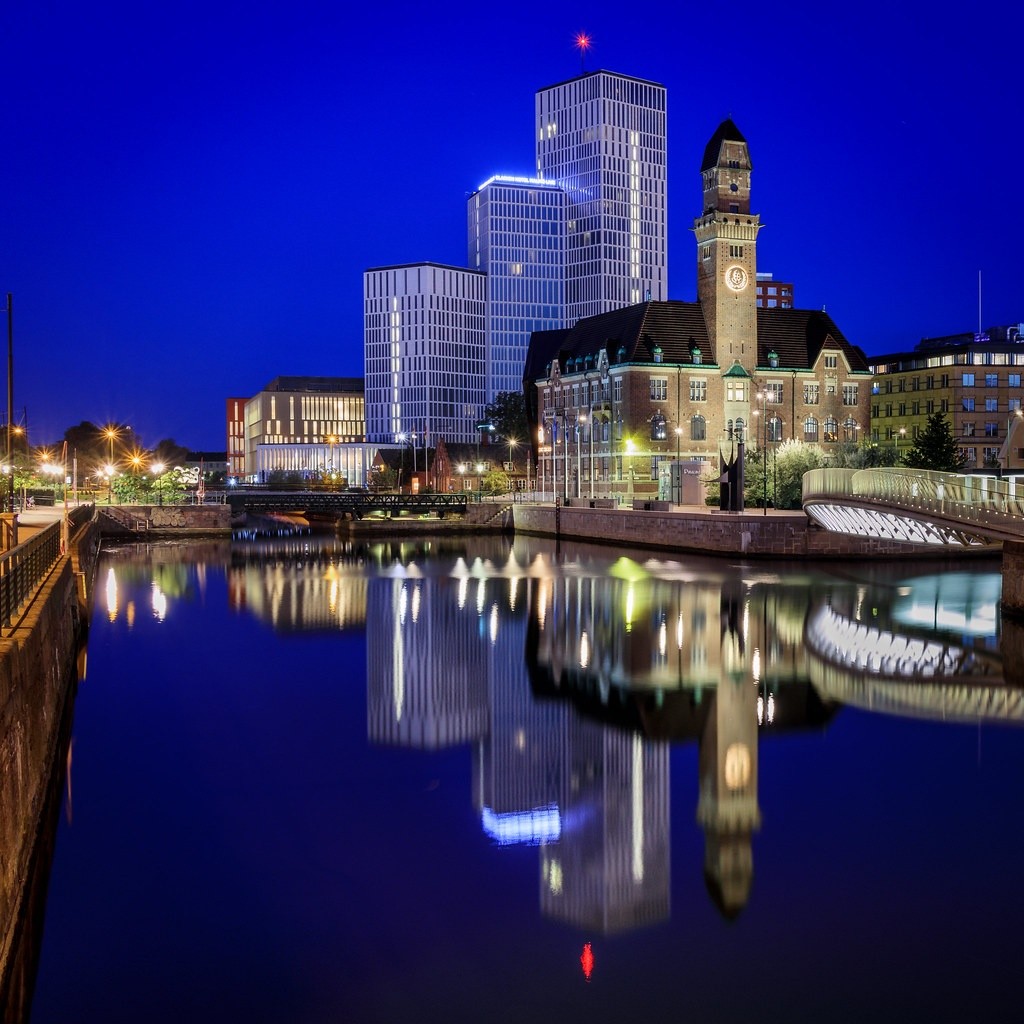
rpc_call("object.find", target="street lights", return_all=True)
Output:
[102,427,119,465]
[458,464,466,494]
[504,437,519,461]
[757,387,775,516]
[326,433,338,469]
[477,464,484,503]
[1007,409,1023,468]
[891,428,906,450]
[659,418,680,508]
[623,438,638,492]
[132,455,141,501]
[397,432,405,485]
[151,461,165,506]
[752,406,780,510]
[14,427,31,467]
[412,428,418,471]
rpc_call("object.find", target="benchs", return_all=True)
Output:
[594,501,614,509]
